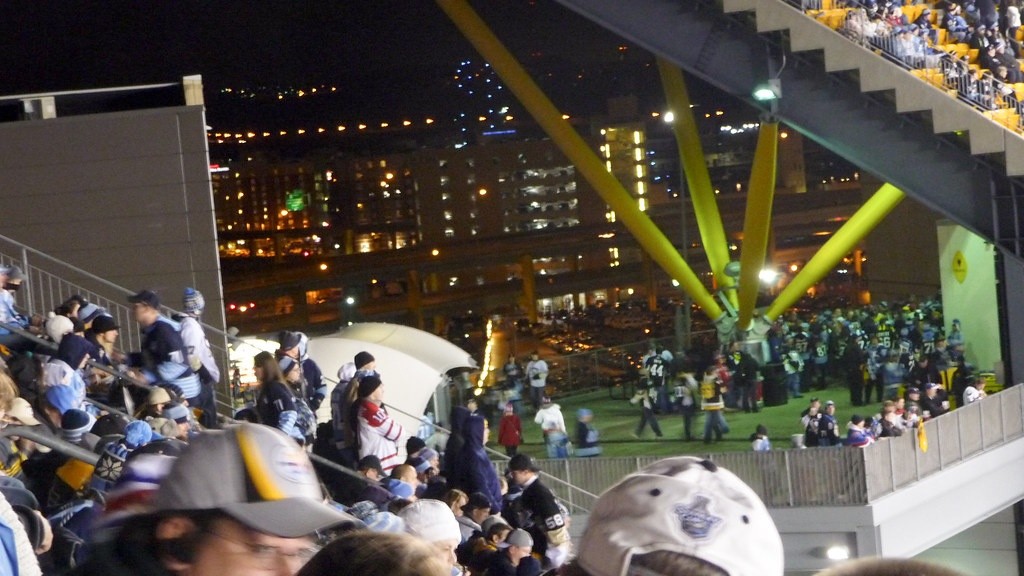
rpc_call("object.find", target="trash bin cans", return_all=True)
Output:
[463,385,493,427]
[762,364,788,407]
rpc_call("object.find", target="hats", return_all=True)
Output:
[419,448,439,460]
[578,456,785,576]
[154,419,356,538]
[398,499,461,544]
[59,409,97,443]
[496,528,533,548]
[838,0,1024,104]
[508,453,542,473]
[411,456,430,474]
[359,455,387,478]
[87,420,153,495]
[87,452,179,527]
[345,499,405,535]
[0,256,594,420]
[406,436,425,455]
[90,413,127,439]
[126,437,189,458]
[460,492,491,511]
[628,298,991,445]
[5,398,42,427]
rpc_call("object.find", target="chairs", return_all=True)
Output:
[806,0,1024,139]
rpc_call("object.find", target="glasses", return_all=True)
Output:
[202,526,319,572]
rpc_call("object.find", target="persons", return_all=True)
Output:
[793,0,1024,126]
[769,293,988,446]
[0,265,782,576]
